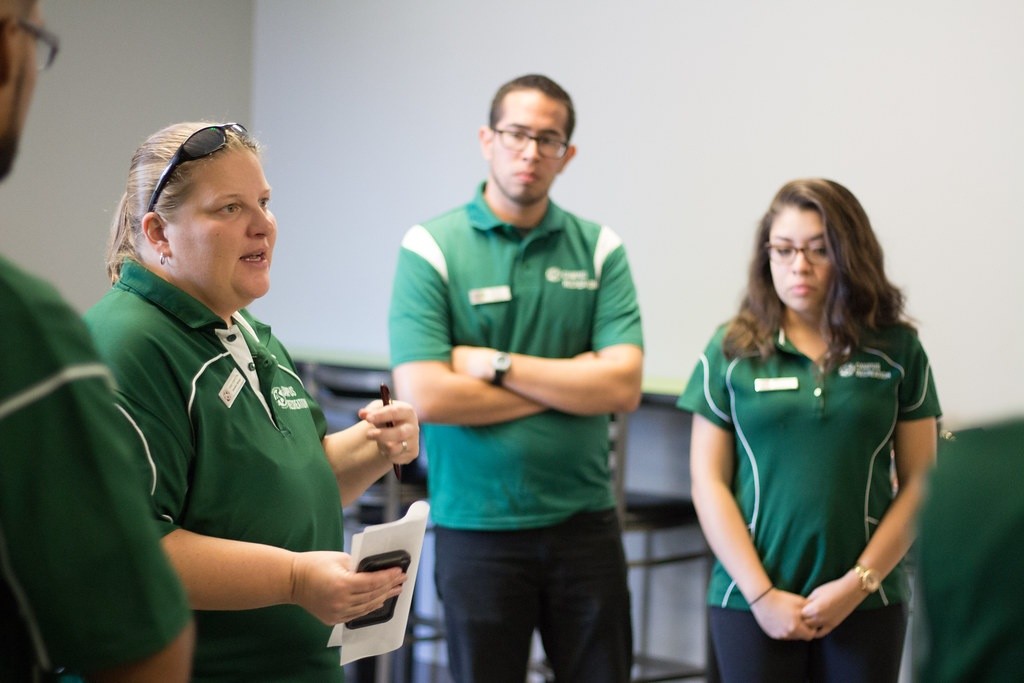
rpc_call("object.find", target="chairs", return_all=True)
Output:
[604,391,723,682]
[284,358,457,682]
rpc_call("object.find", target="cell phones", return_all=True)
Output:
[345,550,411,630]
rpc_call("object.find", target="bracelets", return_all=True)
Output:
[748,584,773,606]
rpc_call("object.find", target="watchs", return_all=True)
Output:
[493,350,514,385]
[854,565,882,595]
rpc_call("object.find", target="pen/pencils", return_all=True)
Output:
[377,384,405,481]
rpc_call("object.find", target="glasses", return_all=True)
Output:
[764,243,832,267]
[493,128,570,161]
[16,20,61,69]
[145,123,249,216]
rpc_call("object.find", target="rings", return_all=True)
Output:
[401,440,408,455]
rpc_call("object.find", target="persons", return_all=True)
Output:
[678,180,944,683]
[1,0,196,682]
[69,121,424,682]
[389,73,634,683]
